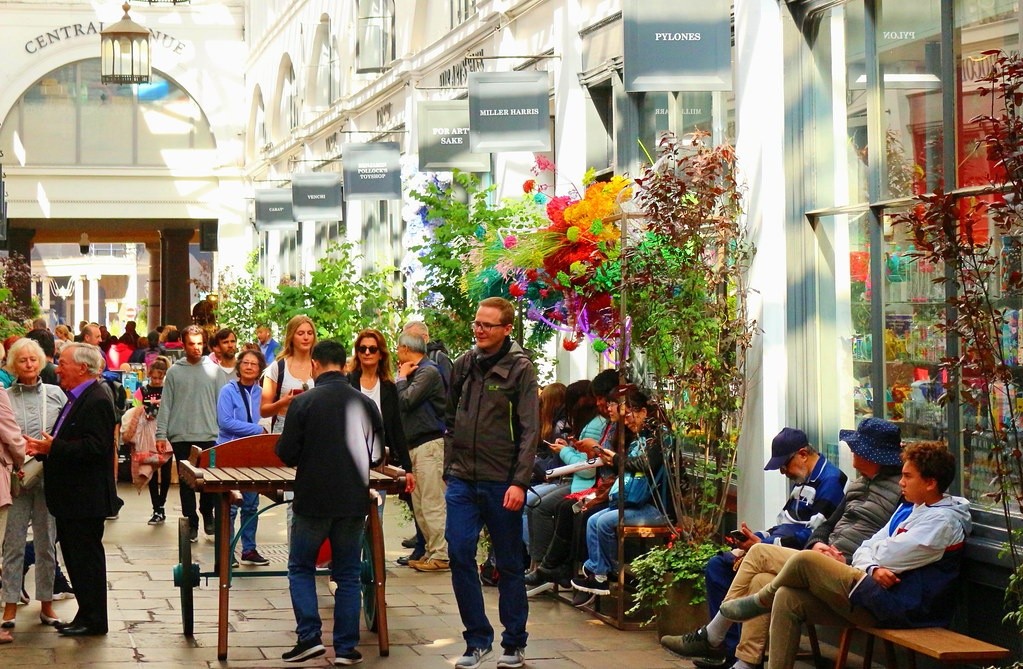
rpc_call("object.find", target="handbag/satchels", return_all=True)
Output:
[19,454,45,490]
[10,471,21,499]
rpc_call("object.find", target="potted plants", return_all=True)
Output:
[613,240,754,642]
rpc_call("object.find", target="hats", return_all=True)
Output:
[765,427,809,470]
[839,416,904,465]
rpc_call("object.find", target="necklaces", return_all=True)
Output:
[295,357,309,371]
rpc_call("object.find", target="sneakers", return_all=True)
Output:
[408,555,429,568]
[0,557,30,604]
[455,645,495,669]
[661,625,728,660]
[572,590,595,608]
[232,554,240,567]
[570,574,610,595]
[414,558,451,572]
[240,550,270,565]
[282,637,326,662]
[52,574,76,600]
[496,648,526,668]
[334,649,362,666]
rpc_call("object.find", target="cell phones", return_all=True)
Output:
[292,389,305,395]
[543,440,552,446]
[730,530,750,543]
[567,436,578,446]
[592,445,609,457]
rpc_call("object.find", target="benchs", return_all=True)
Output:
[557,526,676,632]
[797,615,1010,669]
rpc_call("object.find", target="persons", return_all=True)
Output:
[157,300,315,569]
[276,339,386,665]
[0,338,124,642]
[328,329,415,596]
[395,321,454,571]
[23,317,187,524]
[479,369,690,607]
[442,297,538,669]
[661,418,972,669]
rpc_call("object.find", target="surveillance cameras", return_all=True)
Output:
[79,232,91,253]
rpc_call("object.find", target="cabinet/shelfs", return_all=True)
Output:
[851,280,1023,472]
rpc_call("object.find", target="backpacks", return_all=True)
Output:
[608,436,665,511]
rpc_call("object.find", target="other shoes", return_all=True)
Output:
[189,516,199,542]
[397,553,421,566]
[719,592,771,622]
[525,582,554,597]
[199,506,215,534]
[1,618,16,629]
[525,568,560,585]
[40,611,67,626]
[401,535,418,548]
[148,514,166,525]
[561,570,573,588]
[692,649,738,669]
[55,617,101,636]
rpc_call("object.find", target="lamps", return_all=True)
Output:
[100,2,152,86]
[79,232,90,254]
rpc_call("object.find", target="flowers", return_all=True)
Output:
[458,155,635,364]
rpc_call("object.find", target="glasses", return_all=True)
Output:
[358,346,380,354]
[780,452,796,470]
[471,321,505,332]
[606,402,620,408]
